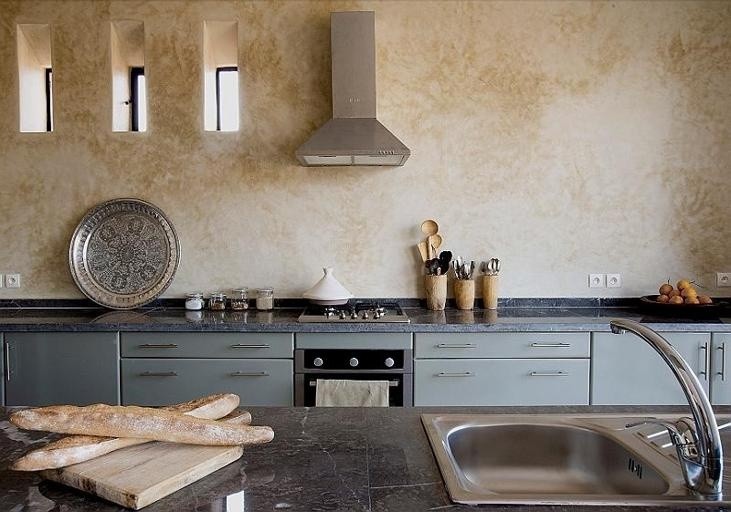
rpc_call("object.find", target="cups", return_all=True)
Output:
[482,275,499,309]
[453,279,475,311]
[425,274,448,311]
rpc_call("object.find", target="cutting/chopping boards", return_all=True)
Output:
[37,442,245,512]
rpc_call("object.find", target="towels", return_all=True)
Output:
[312,376,392,408]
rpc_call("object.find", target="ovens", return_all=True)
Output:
[293,349,413,407]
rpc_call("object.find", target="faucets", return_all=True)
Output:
[608,319,724,495]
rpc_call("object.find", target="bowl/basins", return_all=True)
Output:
[640,294,728,318]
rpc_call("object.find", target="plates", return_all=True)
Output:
[68,199,180,310]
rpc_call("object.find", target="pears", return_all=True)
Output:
[656,277,714,306]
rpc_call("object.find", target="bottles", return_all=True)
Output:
[256,312,274,330]
[186,292,206,310]
[208,290,226,311]
[208,312,225,325]
[231,288,249,311]
[303,265,354,306]
[184,311,205,324]
[232,313,248,325]
[255,287,275,311]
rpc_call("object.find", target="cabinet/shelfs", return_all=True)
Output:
[588,306,731,414]
[0,327,123,413]
[410,319,593,417]
[119,323,297,413]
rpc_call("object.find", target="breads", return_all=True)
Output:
[7,391,275,472]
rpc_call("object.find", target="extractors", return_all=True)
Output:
[295,13,410,167]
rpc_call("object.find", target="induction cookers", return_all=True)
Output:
[297,303,412,324]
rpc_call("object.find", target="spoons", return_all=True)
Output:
[429,251,453,275]
[488,258,502,276]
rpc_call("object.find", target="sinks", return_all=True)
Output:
[420,412,672,507]
